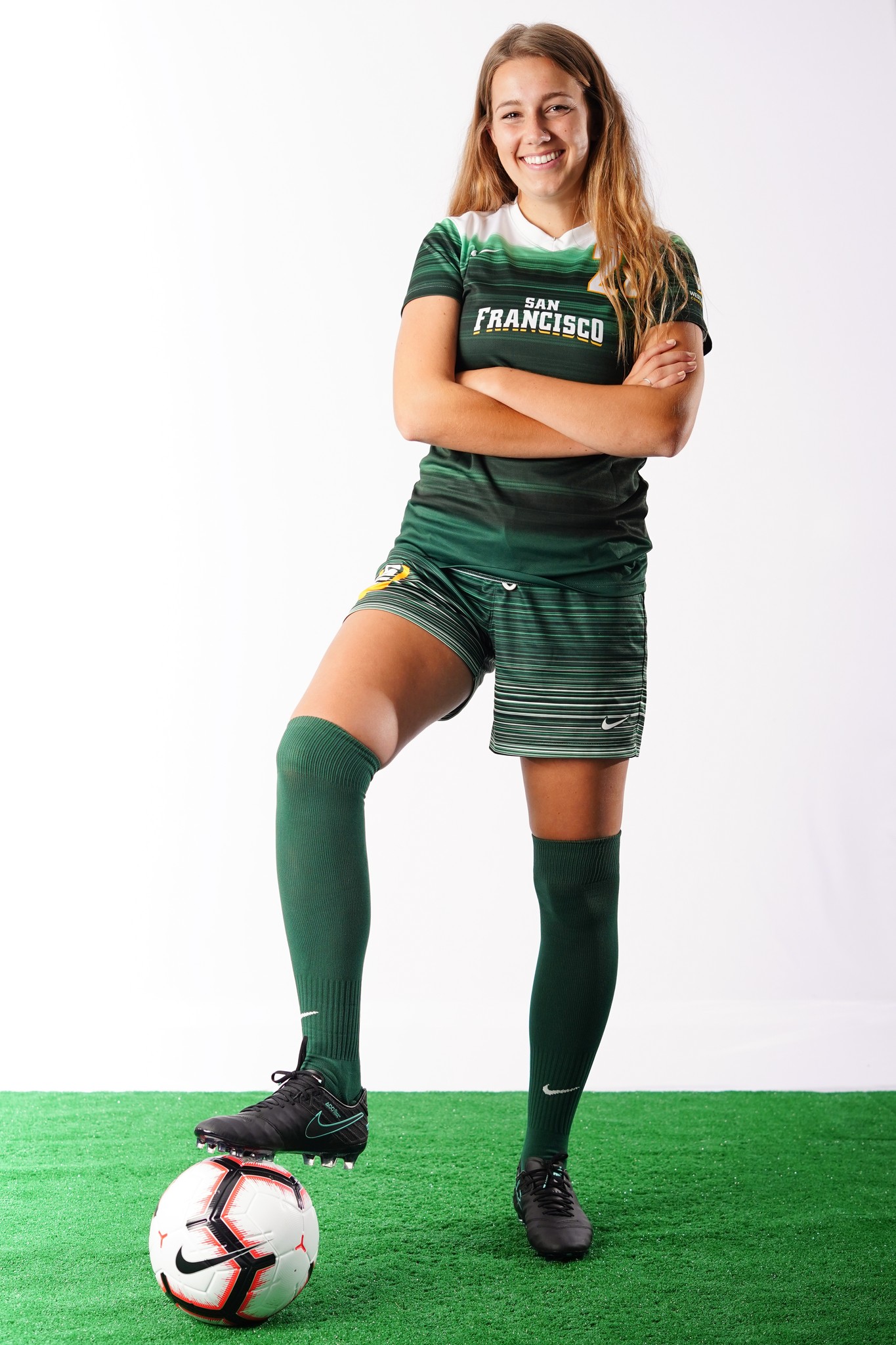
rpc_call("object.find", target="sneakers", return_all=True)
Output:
[194,1035,368,1170]
[512,1152,594,1262]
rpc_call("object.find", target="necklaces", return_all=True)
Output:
[547,233,561,241]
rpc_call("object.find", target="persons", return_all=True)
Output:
[192,22,712,1260]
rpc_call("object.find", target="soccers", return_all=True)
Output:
[147,1154,322,1332]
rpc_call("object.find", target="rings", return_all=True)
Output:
[643,378,653,386]
